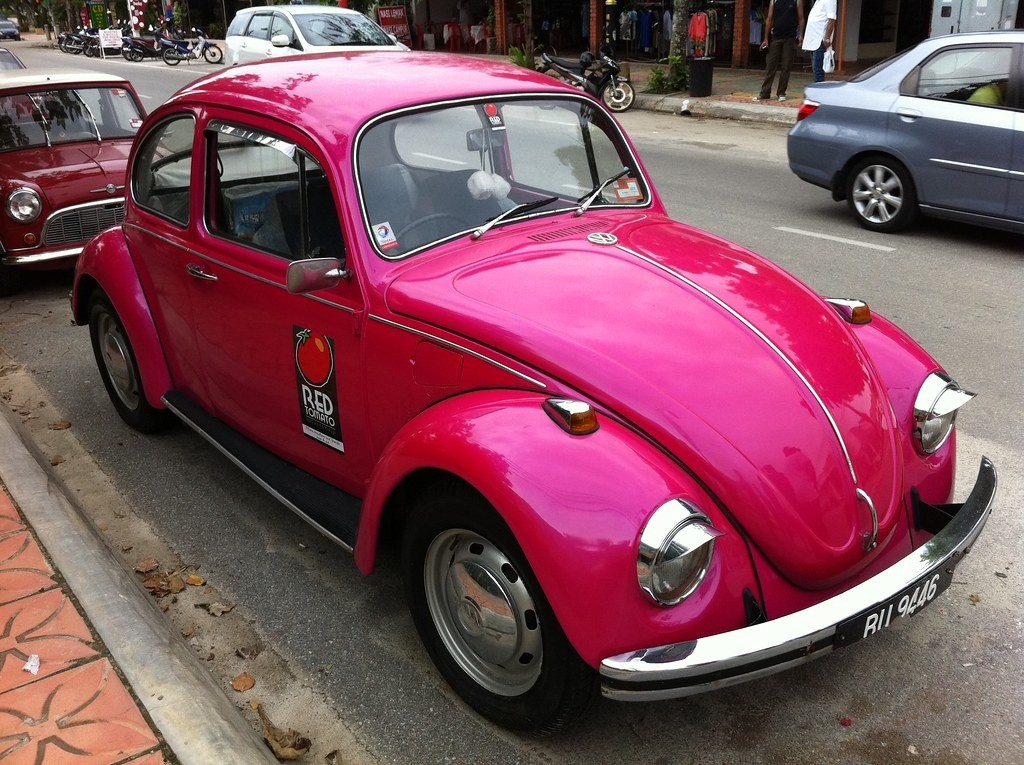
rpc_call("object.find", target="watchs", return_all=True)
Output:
[824,38,830,43]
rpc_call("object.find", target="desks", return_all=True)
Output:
[469,26,484,44]
[507,23,520,44]
[443,24,451,44]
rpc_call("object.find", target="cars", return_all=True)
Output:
[68,50,999,738]
[0,68,194,299]
[223,2,412,74]
[0,48,51,124]
[0,21,21,42]
[785,30,1024,238]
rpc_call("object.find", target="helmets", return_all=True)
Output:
[580,52,597,68]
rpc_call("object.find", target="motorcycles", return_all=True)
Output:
[55,24,221,68]
[535,45,636,113]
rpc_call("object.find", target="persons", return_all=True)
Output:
[802,0,837,82]
[753,0,804,101]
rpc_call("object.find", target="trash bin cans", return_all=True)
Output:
[689,57,714,97]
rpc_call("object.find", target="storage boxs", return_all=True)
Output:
[223,180,308,239]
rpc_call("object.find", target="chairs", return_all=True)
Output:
[415,20,525,55]
[361,163,425,250]
[51,109,90,139]
[420,169,503,247]
[277,177,345,260]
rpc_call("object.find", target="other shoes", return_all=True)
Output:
[778,94,786,102]
[752,96,770,101]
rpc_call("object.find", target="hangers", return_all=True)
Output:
[622,3,765,15]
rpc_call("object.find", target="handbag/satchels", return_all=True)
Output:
[823,43,835,73]
